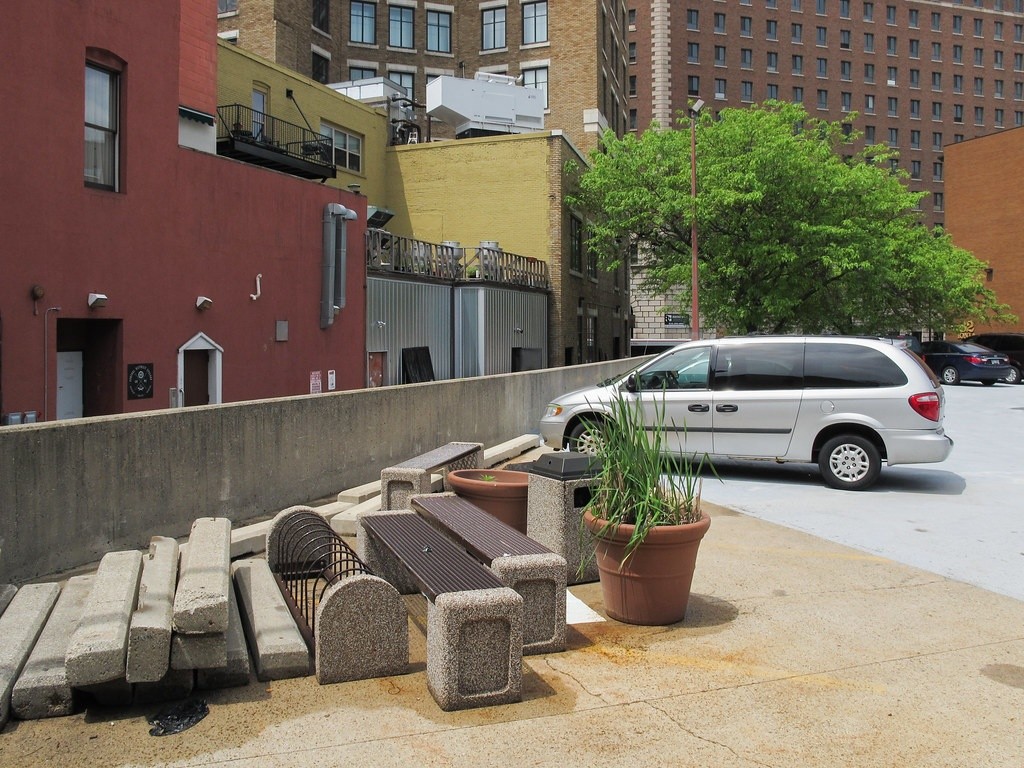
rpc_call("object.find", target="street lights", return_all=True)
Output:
[688,98,707,342]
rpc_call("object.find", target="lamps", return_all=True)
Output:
[196,295,213,311]
[984,267,993,273]
[88,292,108,307]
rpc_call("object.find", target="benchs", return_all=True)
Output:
[409,493,568,658]
[354,511,524,711]
[380,440,485,509]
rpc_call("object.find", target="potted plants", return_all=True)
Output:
[573,368,710,626]
[447,468,530,530]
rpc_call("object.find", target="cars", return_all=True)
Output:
[863,335,925,363]
[659,352,796,383]
[917,341,1011,386]
[544,335,954,491]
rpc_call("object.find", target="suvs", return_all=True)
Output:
[959,332,1024,384]
